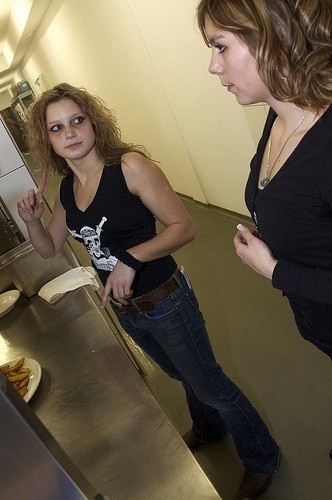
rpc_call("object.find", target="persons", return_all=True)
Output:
[196,0,332,359]
[16,83,285,500]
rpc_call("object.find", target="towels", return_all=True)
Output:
[38,266,99,304]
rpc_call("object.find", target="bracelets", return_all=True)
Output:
[114,248,144,272]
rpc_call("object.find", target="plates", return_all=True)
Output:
[0,290,20,318]
[0,357,42,403]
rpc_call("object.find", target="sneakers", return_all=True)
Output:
[181,429,228,452]
[237,451,281,500]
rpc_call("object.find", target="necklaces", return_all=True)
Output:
[260,107,310,188]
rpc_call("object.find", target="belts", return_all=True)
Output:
[107,266,184,314]
